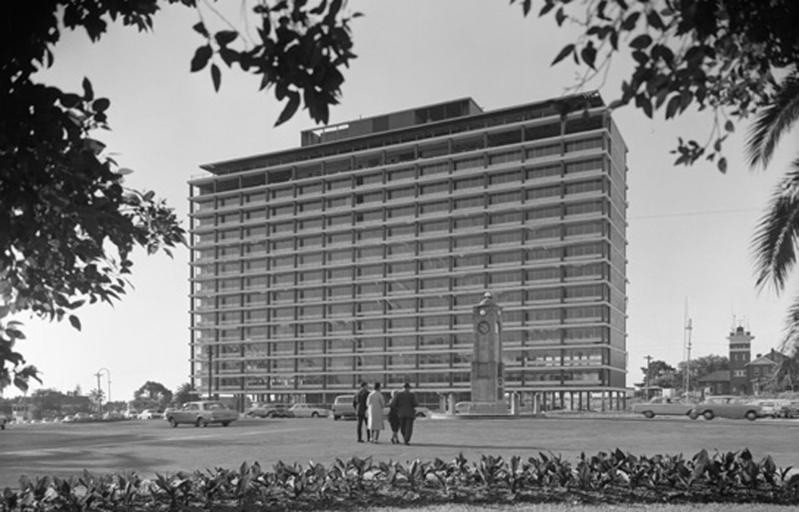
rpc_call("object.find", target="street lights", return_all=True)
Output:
[685,318,693,391]
[93,371,105,415]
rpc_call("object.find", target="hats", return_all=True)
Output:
[403,384,411,388]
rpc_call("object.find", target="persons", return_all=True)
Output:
[352,381,372,442]
[366,381,385,444]
[387,389,400,444]
[397,382,419,445]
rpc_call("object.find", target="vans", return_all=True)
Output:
[332,395,359,421]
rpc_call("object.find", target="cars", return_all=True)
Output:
[289,403,330,418]
[163,400,238,428]
[383,402,433,419]
[7,407,178,426]
[627,391,799,421]
[244,402,294,419]
